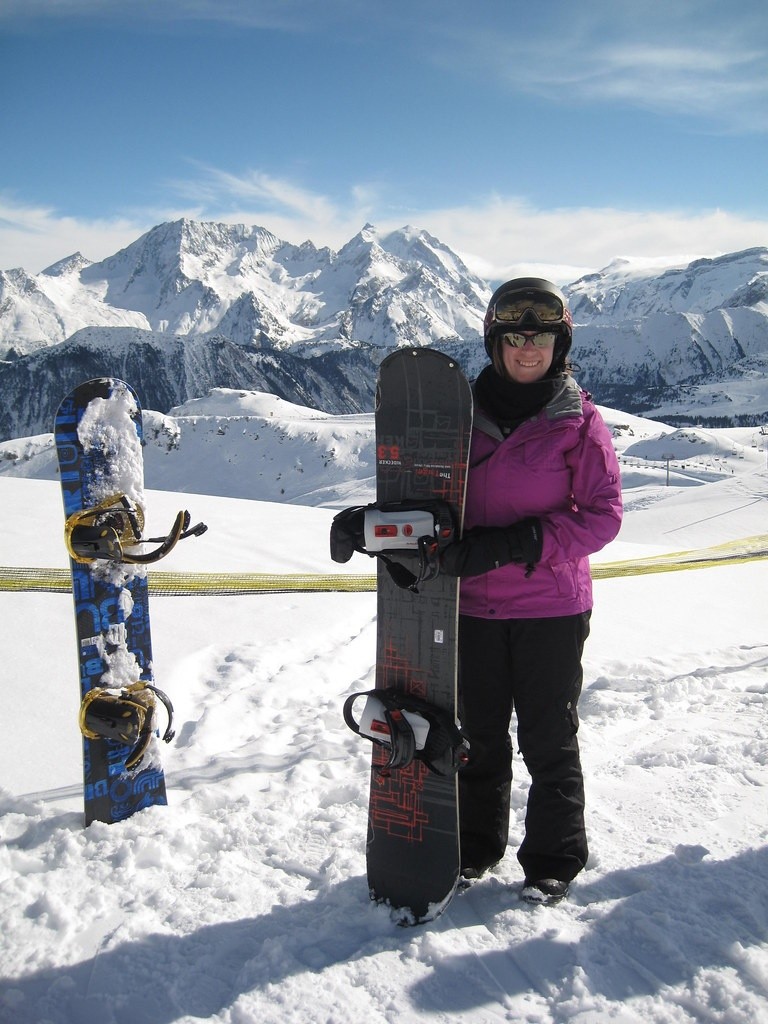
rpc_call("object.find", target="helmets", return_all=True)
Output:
[483,277,573,364]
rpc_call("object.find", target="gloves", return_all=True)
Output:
[330,502,376,564]
[437,516,543,578]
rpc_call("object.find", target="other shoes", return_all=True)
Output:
[521,875,569,904]
[455,860,501,890]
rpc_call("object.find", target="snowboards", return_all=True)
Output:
[50,374,170,828]
[335,344,478,929]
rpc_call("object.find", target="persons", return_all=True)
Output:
[329,272,627,913]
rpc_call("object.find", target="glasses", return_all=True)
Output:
[491,287,566,327]
[500,331,557,348]
